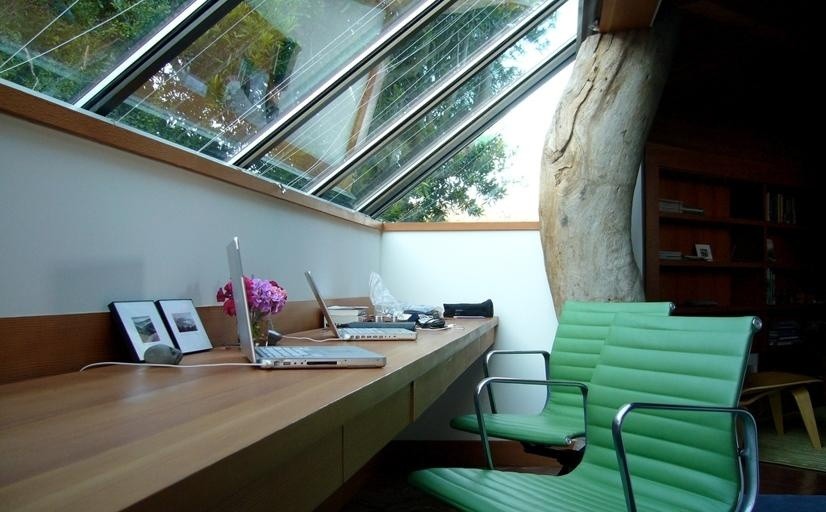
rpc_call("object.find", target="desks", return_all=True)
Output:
[0,296,499,512]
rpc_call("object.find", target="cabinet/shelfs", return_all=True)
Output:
[644,121,817,353]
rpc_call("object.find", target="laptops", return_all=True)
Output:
[226,237,387,369]
[305,270,417,341]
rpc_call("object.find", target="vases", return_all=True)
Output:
[237,320,271,347]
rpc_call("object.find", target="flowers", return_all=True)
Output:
[217,275,289,346]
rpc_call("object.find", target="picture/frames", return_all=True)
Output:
[107,299,214,362]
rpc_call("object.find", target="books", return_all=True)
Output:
[327,306,369,316]
[657,249,705,263]
[657,198,706,217]
[762,190,801,348]
